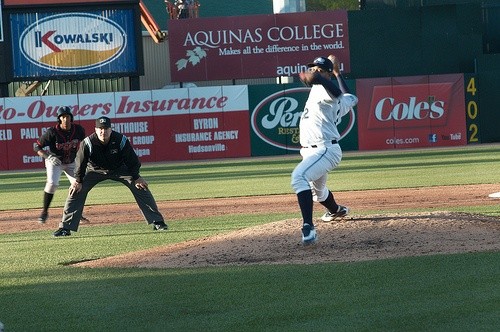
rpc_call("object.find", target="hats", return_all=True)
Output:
[96,115,111,126]
[307,55,333,72]
[58,106,73,118]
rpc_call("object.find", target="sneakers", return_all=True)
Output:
[321,204,349,221]
[300,222,318,244]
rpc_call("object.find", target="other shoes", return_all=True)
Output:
[80,215,89,221]
[39,211,48,222]
[54,227,71,235]
[154,223,168,229]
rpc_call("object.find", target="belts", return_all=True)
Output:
[302,139,337,148]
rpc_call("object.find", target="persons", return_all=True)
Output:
[55,116,168,237]
[33,106,89,223]
[291,56,359,243]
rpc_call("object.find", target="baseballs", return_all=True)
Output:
[327,55,336,65]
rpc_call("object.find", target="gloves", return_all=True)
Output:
[69,180,82,196]
[47,154,62,165]
[135,176,149,190]
